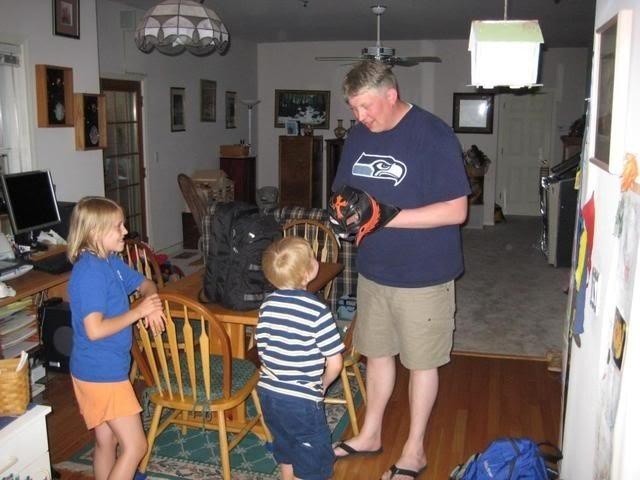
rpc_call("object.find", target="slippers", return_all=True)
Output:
[379,463,428,480]
[330,440,383,458]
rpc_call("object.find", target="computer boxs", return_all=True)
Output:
[39,302,71,373]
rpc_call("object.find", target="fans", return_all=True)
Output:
[313,3,444,70]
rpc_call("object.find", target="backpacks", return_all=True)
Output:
[198,196,284,310]
[450,436,562,480]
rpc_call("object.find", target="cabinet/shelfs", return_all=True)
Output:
[277,135,321,209]
[220,156,257,201]
[0,402,58,480]
[324,139,342,208]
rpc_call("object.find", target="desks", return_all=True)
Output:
[128,256,343,409]
[1,243,76,401]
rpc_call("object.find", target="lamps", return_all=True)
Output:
[132,0,230,56]
[239,99,261,154]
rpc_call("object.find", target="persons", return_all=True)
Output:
[254,235,344,480]
[328,60,471,480]
[65,195,170,479]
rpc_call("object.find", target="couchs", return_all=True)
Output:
[199,208,357,300]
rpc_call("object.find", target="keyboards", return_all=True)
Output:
[31,251,73,275]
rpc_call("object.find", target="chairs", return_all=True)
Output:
[248,220,341,350]
[119,241,208,384]
[176,173,210,267]
[321,305,368,435]
[134,294,275,480]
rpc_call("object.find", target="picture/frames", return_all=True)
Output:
[225,91,237,129]
[200,78,217,122]
[285,119,299,137]
[170,86,187,133]
[452,92,495,134]
[274,88,332,130]
[588,9,632,176]
[52,0,81,41]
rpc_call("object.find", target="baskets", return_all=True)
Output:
[220,144,249,158]
[0,359,30,416]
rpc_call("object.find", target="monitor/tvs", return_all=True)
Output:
[0,169,61,251]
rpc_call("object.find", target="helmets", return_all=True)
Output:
[328,184,400,245]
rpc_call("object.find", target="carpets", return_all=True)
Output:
[50,336,368,480]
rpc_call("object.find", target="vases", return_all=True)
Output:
[334,119,346,138]
[346,120,356,136]
